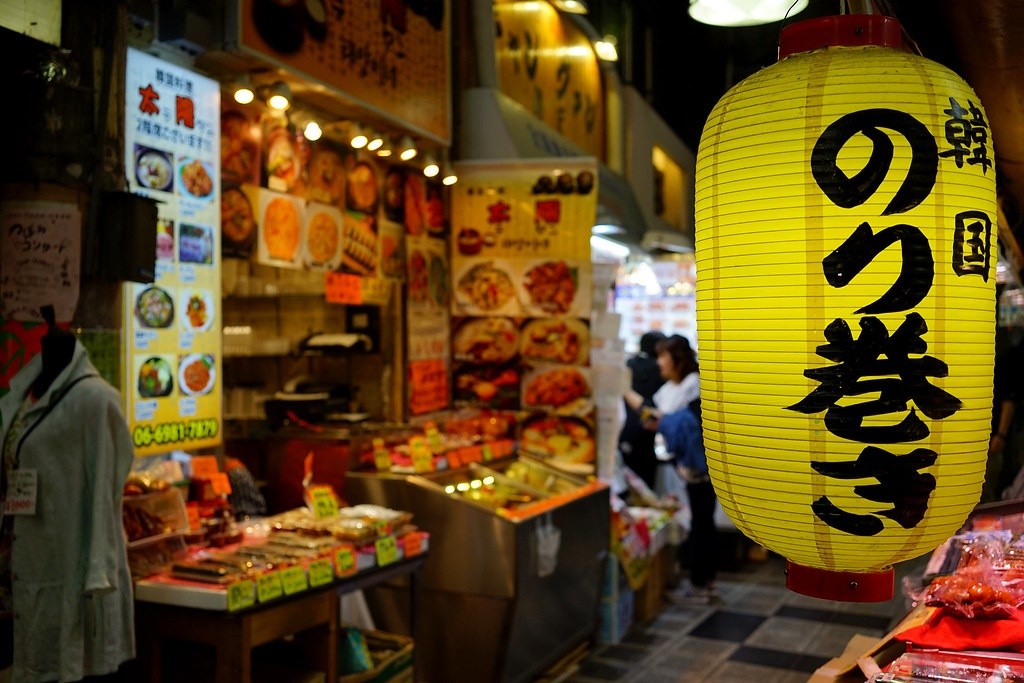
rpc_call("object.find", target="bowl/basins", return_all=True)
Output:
[263,129,301,191]
[454,258,518,411]
[383,167,404,217]
[140,357,173,399]
[305,149,347,205]
[347,160,378,213]
[137,287,174,329]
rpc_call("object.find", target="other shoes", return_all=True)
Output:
[732,554,768,573]
[666,588,711,605]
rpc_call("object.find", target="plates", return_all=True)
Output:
[520,319,590,367]
[178,353,215,397]
[179,289,214,332]
[522,366,595,416]
[519,259,589,316]
[256,189,303,268]
[302,202,344,273]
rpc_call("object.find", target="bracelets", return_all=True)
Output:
[998,431,1009,439]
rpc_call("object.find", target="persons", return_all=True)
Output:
[0,305,136,683]
[616,329,722,606]
[989,283,1024,501]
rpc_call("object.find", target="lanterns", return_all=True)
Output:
[694,10,999,603]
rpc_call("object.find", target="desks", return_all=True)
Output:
[135,553,428,683]
